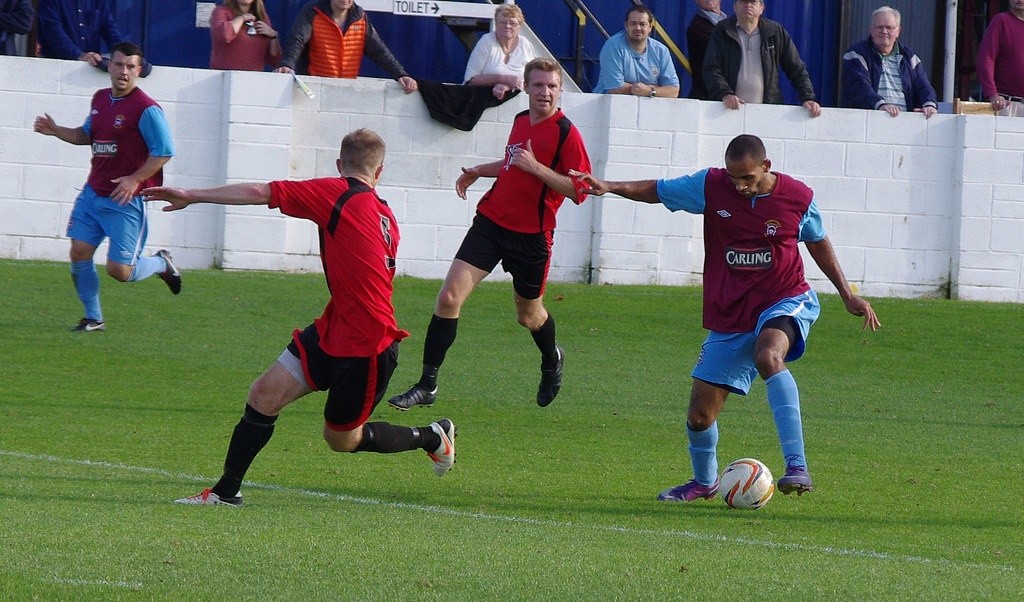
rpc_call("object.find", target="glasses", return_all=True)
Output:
[499,21,519,27]
[875,26,897,33]
[737,0,761,5]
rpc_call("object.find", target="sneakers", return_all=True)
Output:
[174,489,244,507]
[72,318,105,331]
[156,250,182,294]
[657,475,720,502]
[537,345,565,407]
[777,467,813,496]
[388,384,438,411]
[427,418,458,476]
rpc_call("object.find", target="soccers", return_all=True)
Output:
[719,458,776,510]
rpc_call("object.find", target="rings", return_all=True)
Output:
[995,101,999,103]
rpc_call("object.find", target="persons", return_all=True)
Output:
[0,0,36,57]
[698,0,821,118]
[463,5,542,99]
[35,0,124,66]
[33,41,182,334]
[570,134,882,503]
[976,0,1024,118]
[838,5,938,120]
[593,5,680,99]
[685,0,727,100]
[389,57,591,411]
[272,0,417,94]
[209,0,282,72]
[138,127,457,507]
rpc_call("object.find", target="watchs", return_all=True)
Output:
[649,84,656,98]
[271,31,279,39]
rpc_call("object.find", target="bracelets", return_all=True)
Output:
[239,14,245,21]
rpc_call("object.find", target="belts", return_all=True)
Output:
[998,94,1022,102]
